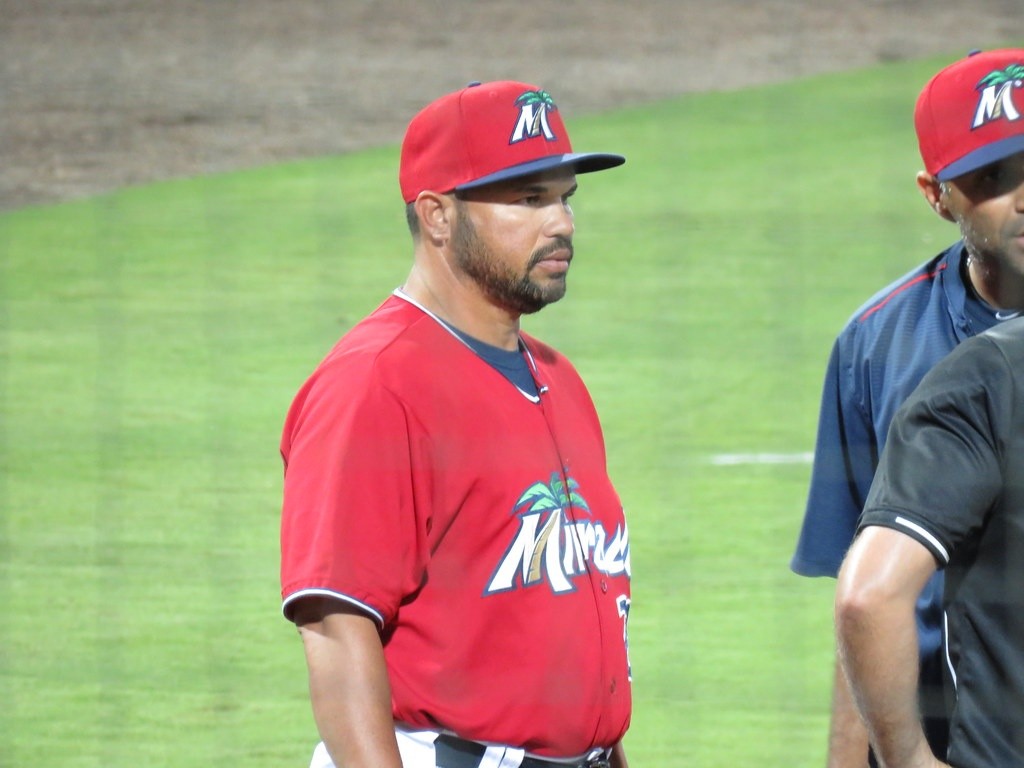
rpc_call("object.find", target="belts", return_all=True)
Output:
[435,734,610,768]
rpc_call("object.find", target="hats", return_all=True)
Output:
[399,80,626,205]
[915,49,1024,183]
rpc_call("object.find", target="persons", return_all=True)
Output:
[280,81,638,768]
[835,315,1024,768]
[788,43,1024,768]
